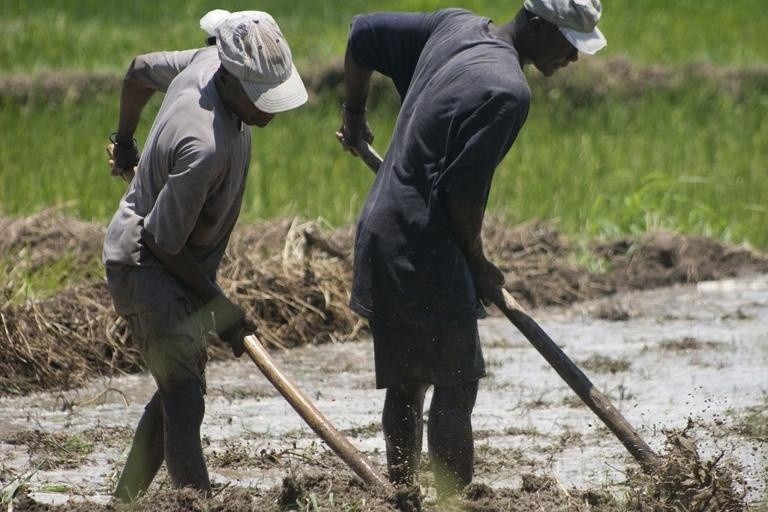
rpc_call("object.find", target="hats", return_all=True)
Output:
[524,0,608,55]
[216,11,308,113]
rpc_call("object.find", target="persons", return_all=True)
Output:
[337,1,606,494]
[97,8,311,504]
